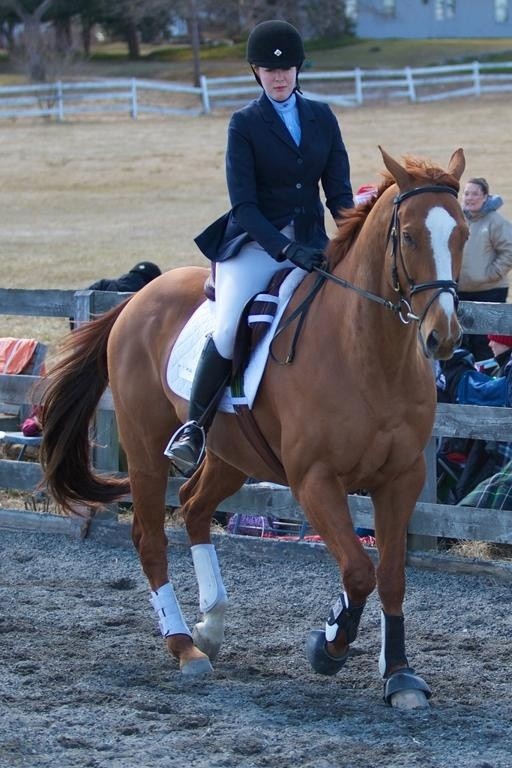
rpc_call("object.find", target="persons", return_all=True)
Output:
[427,334,511,512]
[457,175,512,347]
[157,17,358,468]
[69,262,163,330]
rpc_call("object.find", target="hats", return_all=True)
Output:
[488,335,512,347]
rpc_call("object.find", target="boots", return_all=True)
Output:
[170,332,232,474]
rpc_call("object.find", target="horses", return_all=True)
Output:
[30,145,470,712]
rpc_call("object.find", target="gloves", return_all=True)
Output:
[286,242,324,273]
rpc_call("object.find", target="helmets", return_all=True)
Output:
[129,262,159,279]
[246,20,305,69]
